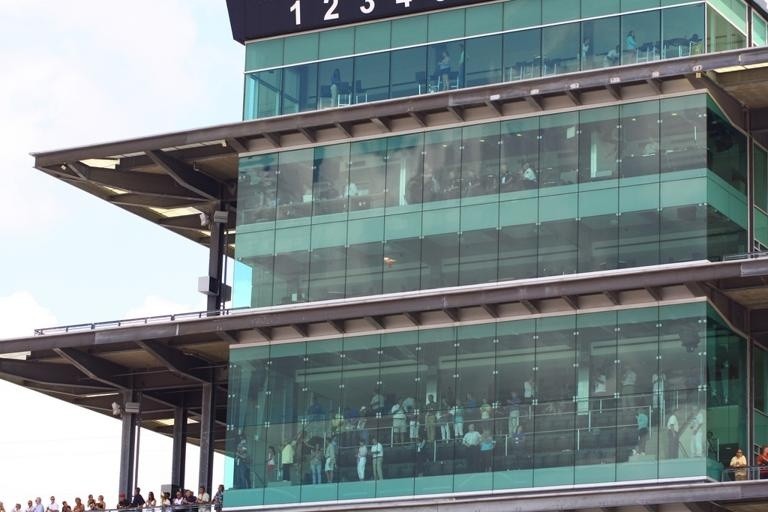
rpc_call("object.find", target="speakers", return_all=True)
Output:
[125,402,141,414]
[214,211,228,223]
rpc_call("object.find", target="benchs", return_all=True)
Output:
[305,407,649,483]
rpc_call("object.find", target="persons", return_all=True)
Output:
[118,492,129,508]
[437,51,451,91]
[756,446,767,479]
[330,68,340,107]
[74,497,85,510]
[47,496,60,510]
[15,503,22,511]
[239,138,705,222]
[62,501,71,511]
[33,497,44,511]
[171,488,198,511]
[28,500,34,510]
[626,30,638,63]
[456,44,469,89]
[195,486,210,511]
[161,492,172,511]
[604,45,620,68]
[87,495,96,510]
[128,487,145,507]
[236,356,728,490]
[576,36,591,71]
[145,492,156,510]
[212,484,224,511]
[92,495,105,510]
[730,448,747,481]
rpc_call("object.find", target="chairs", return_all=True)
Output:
[317,79,367,111]
[635,34,704,64]
[437,166,558,203]
[240,192,373,226]
[504,55,561,81]
[414,69,460,97]
[622,145,715,177]
